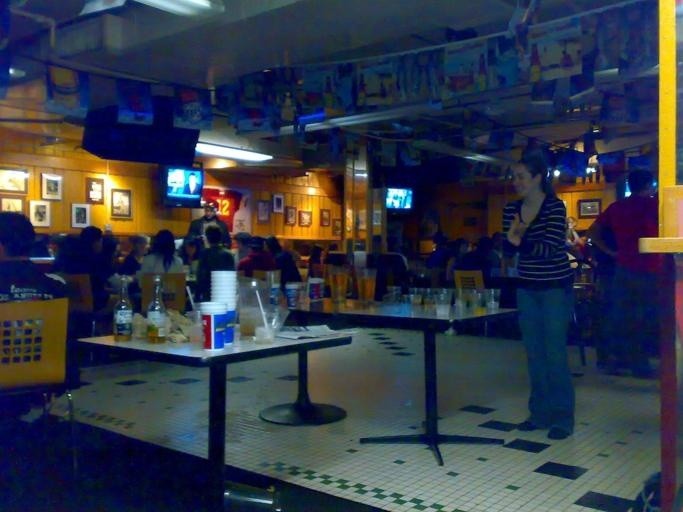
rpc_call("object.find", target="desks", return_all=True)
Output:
[337,303,522,466]
[80,325,353,508]
[255,296,374,426]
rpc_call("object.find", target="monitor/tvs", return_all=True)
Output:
[384,186,413,210]
[159,166,203,207]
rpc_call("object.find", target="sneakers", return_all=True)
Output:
[632,365,658,379]
[598,363,629,376]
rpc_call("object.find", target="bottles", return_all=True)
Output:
[113,274,134,342]
[147,274,168,344]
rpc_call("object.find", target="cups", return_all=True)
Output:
[327,264,379,307]
[387,286,501,317]
[192,270,326,353]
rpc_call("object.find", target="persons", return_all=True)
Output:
[500,159,576,440]
[430,171,662,379]
[1,213,69,412]
[51,205,303,367]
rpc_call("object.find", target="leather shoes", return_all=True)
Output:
[548,426,574,439]
[517,420,550,431]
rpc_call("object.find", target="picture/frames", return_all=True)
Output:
[0,194,26,215]
[41,173,63,201]
[86,177,104,205]
[0,167,29,195]
[30,200,51,229]
[578,199,602,219]
[110,188,133,220]
[255,194,342,235]
[71,202,90,229]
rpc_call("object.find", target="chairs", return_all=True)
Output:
[0,296,77,485]
